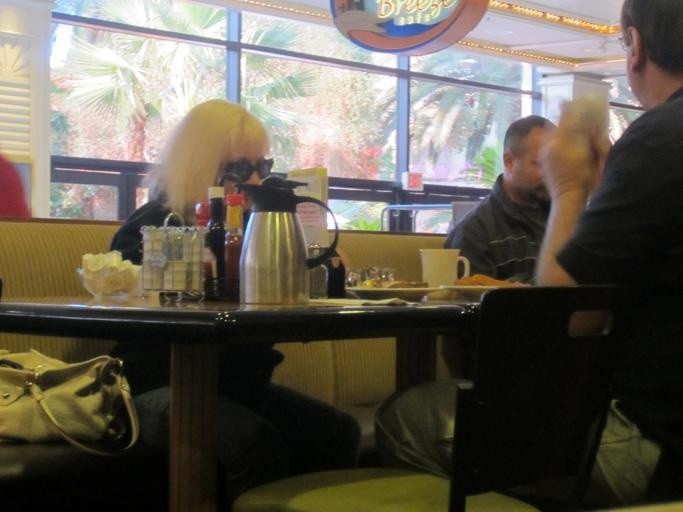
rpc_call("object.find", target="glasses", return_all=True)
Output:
[618,33,631,52]
[221,158,274,181]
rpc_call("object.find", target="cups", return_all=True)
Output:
[420,249,470,298]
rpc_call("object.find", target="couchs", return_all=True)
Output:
[324,228,463,468]
[2,216,397,487]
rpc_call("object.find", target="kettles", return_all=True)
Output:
[235,176,339,304]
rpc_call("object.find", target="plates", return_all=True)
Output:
[346,282,442,300]
[444,284,530,298]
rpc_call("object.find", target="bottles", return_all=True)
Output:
[307,244,329,299]
[202,186,226,299]
[225,193,244,295]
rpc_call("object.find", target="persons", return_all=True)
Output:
[373,0,683,510]
[442,115,557,378]
[110,99,361,489]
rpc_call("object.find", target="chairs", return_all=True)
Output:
[233,278,625,508]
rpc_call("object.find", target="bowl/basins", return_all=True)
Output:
[77,264,142,295]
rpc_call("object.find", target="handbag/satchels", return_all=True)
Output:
[1,347,141,456]
